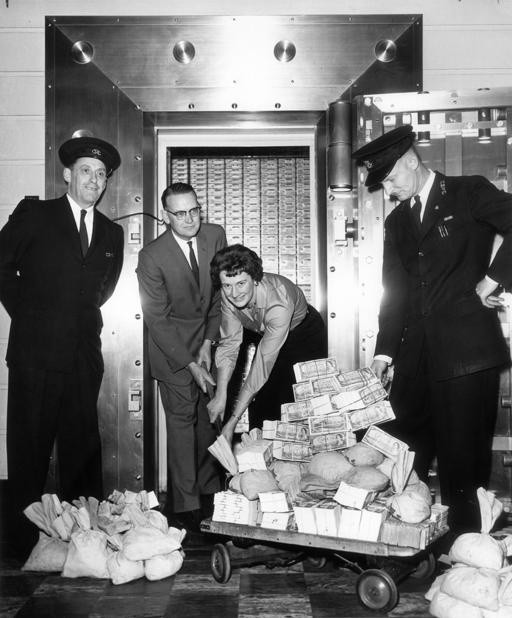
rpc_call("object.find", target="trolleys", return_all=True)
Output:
[200,359,450,613]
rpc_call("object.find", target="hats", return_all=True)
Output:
[351,125,416,187]
[58,130,121,176]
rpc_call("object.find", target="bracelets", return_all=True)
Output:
[232,414,240,420]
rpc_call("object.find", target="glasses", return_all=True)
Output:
[165,201,202,220]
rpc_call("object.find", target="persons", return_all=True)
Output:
[325,360,335,373]
[298,427,309,442]
[135,181,231,531]
[205,243,323,449]
[333,432,345,449]
[349,125,512,555]
[2,136,124,550]
[389,439,401,454]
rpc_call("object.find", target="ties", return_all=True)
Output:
[79,210,88,257]
[187,241,199,286]
[412,195,422,231]
